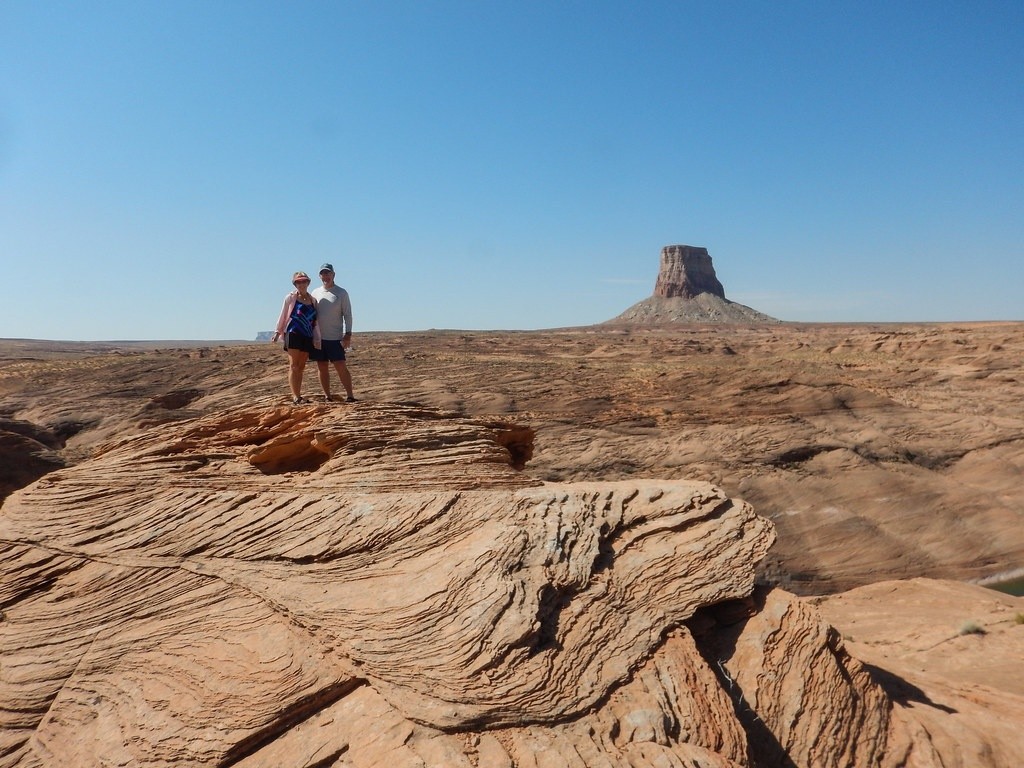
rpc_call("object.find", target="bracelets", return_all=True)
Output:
[346,332,351,336]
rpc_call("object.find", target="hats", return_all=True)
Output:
[319,263,333,273]
[292,272,311,285]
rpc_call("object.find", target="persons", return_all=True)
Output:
[271,272,321,405]
[311,263,357,402]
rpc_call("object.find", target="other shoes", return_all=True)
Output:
[294,397,309,405]
[344,396,355,402]
[324,396,332,402]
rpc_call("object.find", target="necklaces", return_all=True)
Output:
[298,294,308,301]
